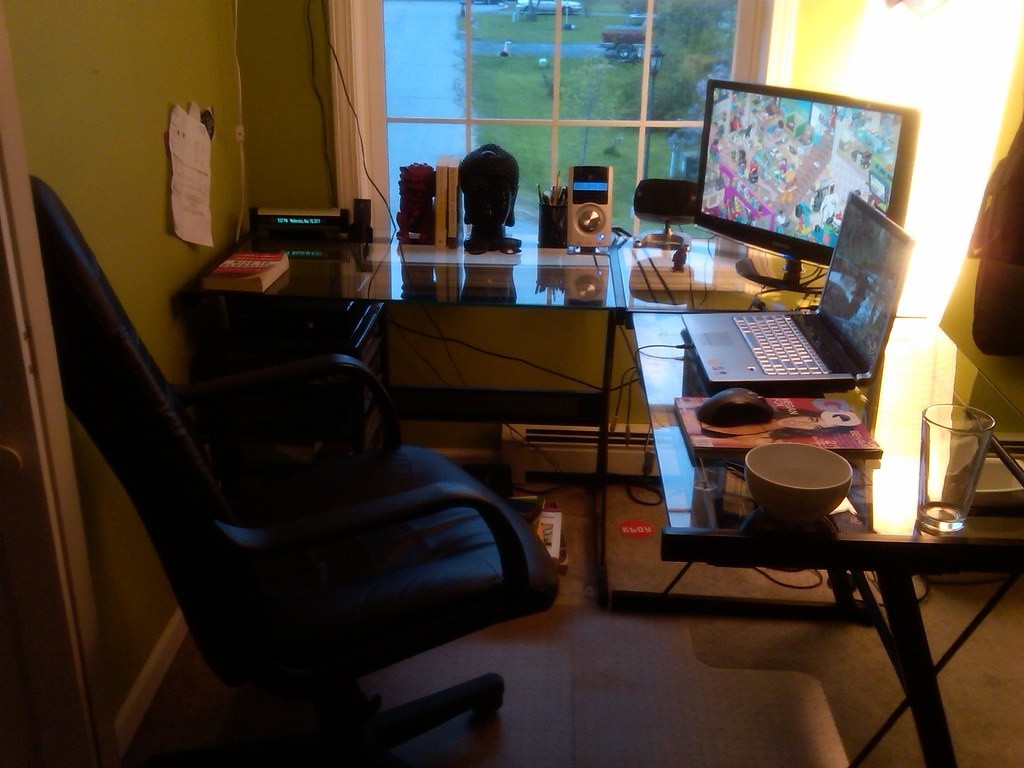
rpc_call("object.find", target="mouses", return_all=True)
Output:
[695,388,774,428]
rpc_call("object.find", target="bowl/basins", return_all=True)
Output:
[745,442,853,520]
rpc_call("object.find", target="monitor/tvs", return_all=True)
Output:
[692,79,921,294]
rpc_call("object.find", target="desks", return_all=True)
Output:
[171,229,1024,768]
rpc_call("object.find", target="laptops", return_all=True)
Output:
[680,193,915,392]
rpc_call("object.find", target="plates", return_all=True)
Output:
[971,458,1024,505]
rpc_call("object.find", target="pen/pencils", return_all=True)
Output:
[537,171,571,206]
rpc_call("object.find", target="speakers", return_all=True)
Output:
[633,179,699,247]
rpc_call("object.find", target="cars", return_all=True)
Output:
[602,11,662,58]
[516,0,583,16]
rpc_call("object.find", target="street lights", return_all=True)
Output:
[642,44,667,181]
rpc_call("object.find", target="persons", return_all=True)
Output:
[678,398,855,446]
[459,144,520,254]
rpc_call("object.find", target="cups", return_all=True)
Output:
[916,404,996,537]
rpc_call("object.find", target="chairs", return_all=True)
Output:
[29,172,562,767]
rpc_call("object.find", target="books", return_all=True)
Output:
[673,396,886,460]
[435,156,463,250]
[205,250,290,293]
